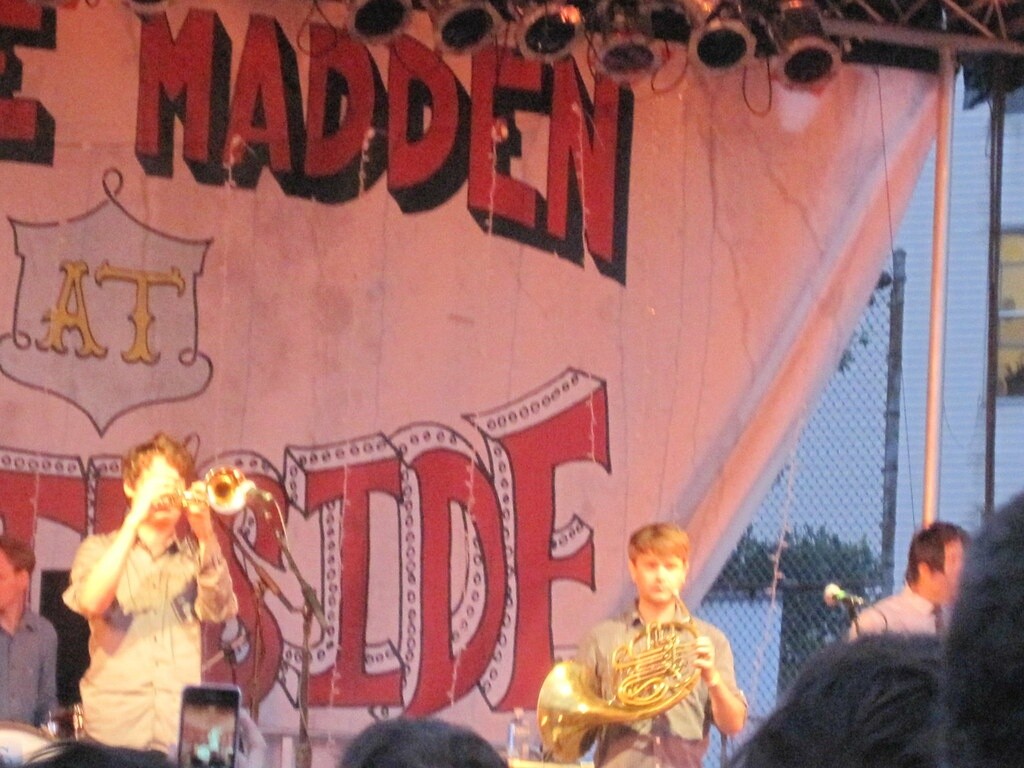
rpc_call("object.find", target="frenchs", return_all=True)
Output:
[530,590,705,764]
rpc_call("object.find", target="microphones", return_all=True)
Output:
[238,479,273,502]
[823,583,864,608]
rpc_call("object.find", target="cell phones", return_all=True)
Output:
[175,684,242,768]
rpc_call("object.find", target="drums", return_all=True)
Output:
[0,719,61,765]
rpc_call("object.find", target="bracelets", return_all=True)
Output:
[706,669,720,688]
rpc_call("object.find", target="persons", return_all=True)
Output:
[842,518,968,646]
[727,631,945,767]
[60,427,239,762]
[936,489,1024,768]
[566,521,749,768]
[0,533,59,731]
[334,716,513,768]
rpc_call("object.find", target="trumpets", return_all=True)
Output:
[159,464,254,517]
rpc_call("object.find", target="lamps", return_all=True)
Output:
[347,0,842,92]
